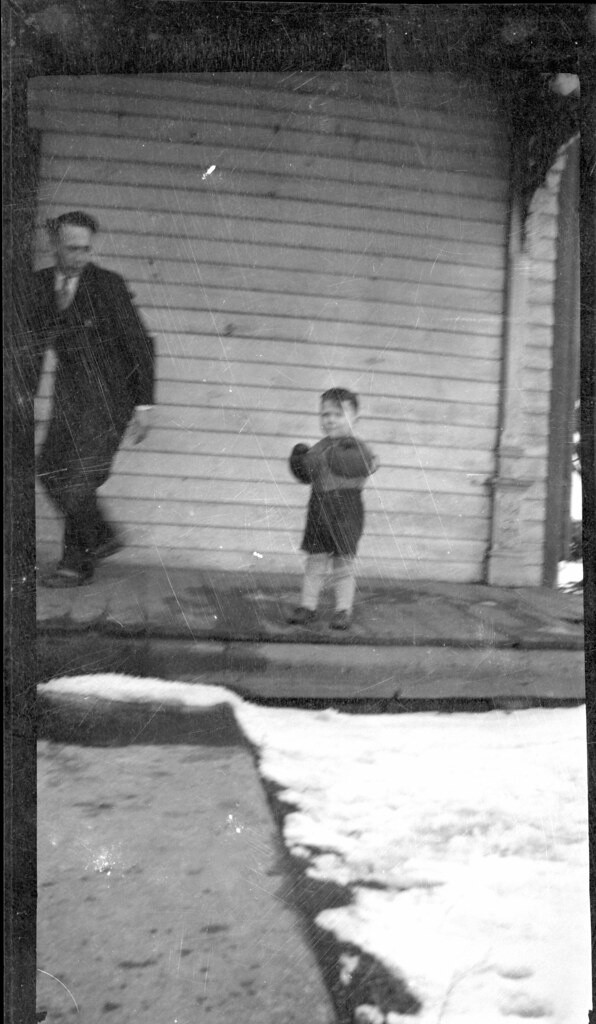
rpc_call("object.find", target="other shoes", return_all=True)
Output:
[288,606,314,624]
[330,611,350,630]
[43,565,92,588]
[81,535,125,562]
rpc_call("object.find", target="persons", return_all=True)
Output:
[286,388,377,630]
[25,211,155,588]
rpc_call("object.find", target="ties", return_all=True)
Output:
[57,275,72,309]
